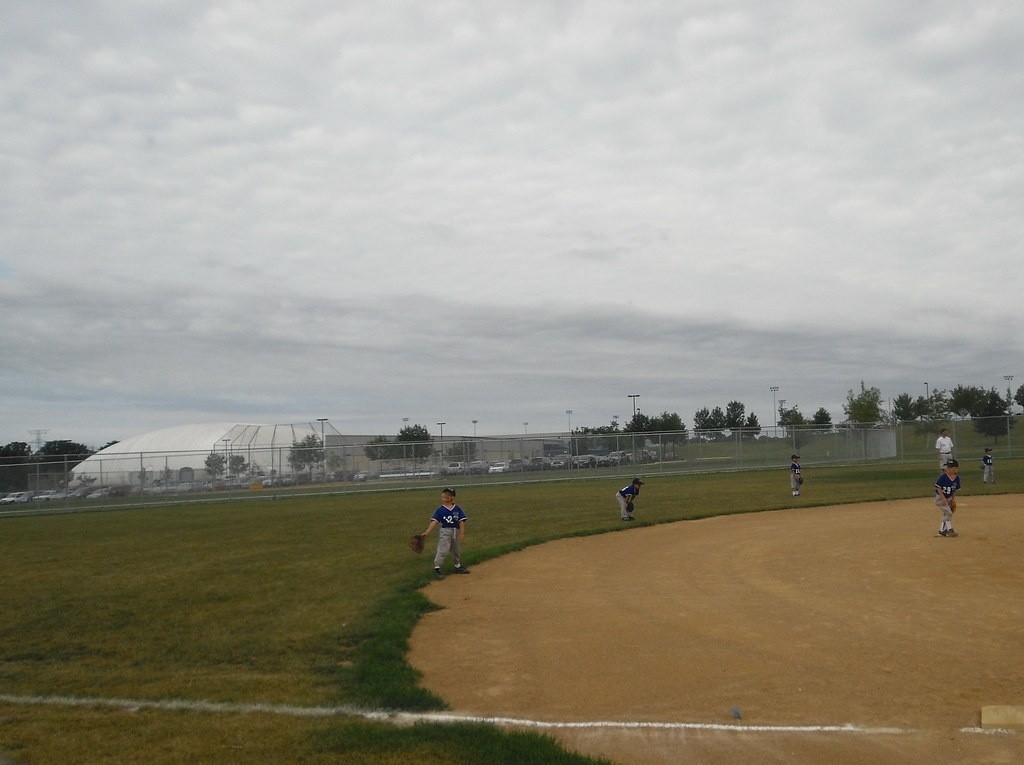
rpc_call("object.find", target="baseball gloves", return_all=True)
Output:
[409,535,424,555]
[799,477,803,484]
[626,501,634,512]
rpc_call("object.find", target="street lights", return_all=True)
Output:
[770,386,779,437]
[637,408,641,421]
[437,423,445,466]
[924,382,928,398]
[222,439,230,476]
[628,394,640,431]
[523,422,528,433]
[317,419,328,474]
[1004,375,1013,409]
[566,410,572,455]
[472,420,477,435]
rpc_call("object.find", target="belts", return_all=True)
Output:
[946,452,950,454]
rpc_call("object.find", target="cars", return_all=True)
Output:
[354,471,368,481]
[443,461,466,474]
[0,474,293,505]
[469,455,608,475]
[631,450,656,463]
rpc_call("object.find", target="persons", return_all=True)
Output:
[790,454,801,496]
[615,477,645,521]
[934,427,954,474]
[934,459,961,537]
[982,448,996,484]
[421,487,470,580]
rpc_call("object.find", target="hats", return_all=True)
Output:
[985,448,992,451]
[944,459,958,467]
[633,478,644,484]
[791,455,800,460]
[443,487,455,497]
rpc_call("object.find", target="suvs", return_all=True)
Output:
[608,451,630,466]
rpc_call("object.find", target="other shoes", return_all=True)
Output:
[432,568,444,580]
[793,492,799,496]
[454,566,470,574]
[939,529,958,537]
